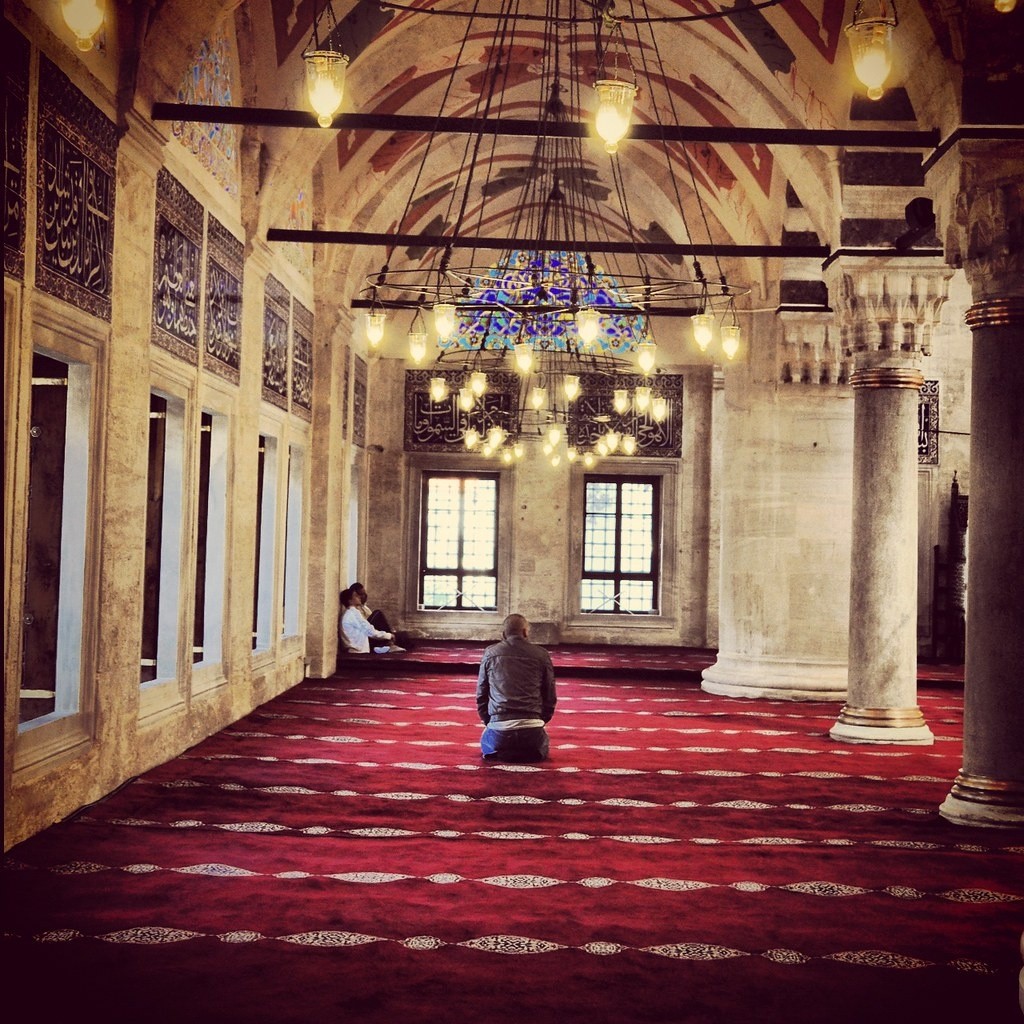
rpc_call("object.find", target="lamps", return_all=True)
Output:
[59,0,105,51]
[351,0,750,466]
[843,0,899,101]
[301,0,350,130]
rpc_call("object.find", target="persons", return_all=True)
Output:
[475,613,559,761]
[339,582,408,654]
